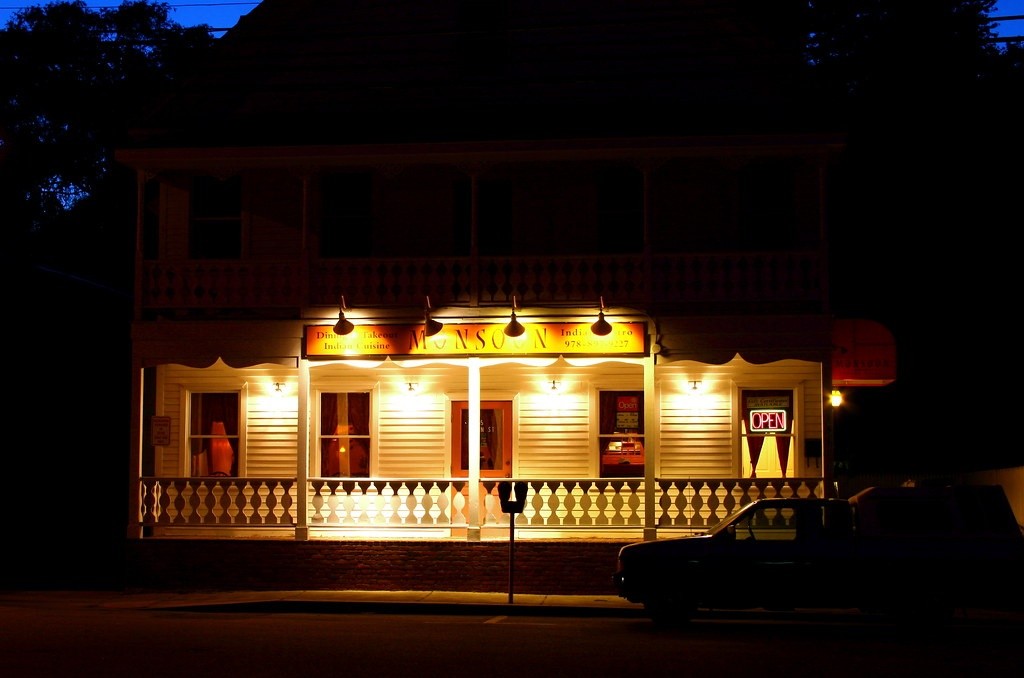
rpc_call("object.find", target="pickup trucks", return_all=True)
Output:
[612,482,1024,645]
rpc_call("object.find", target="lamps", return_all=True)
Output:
[424,295,444,337]
[333,293,355,335]
[502,295,525,337]
[591,295,613,336]
